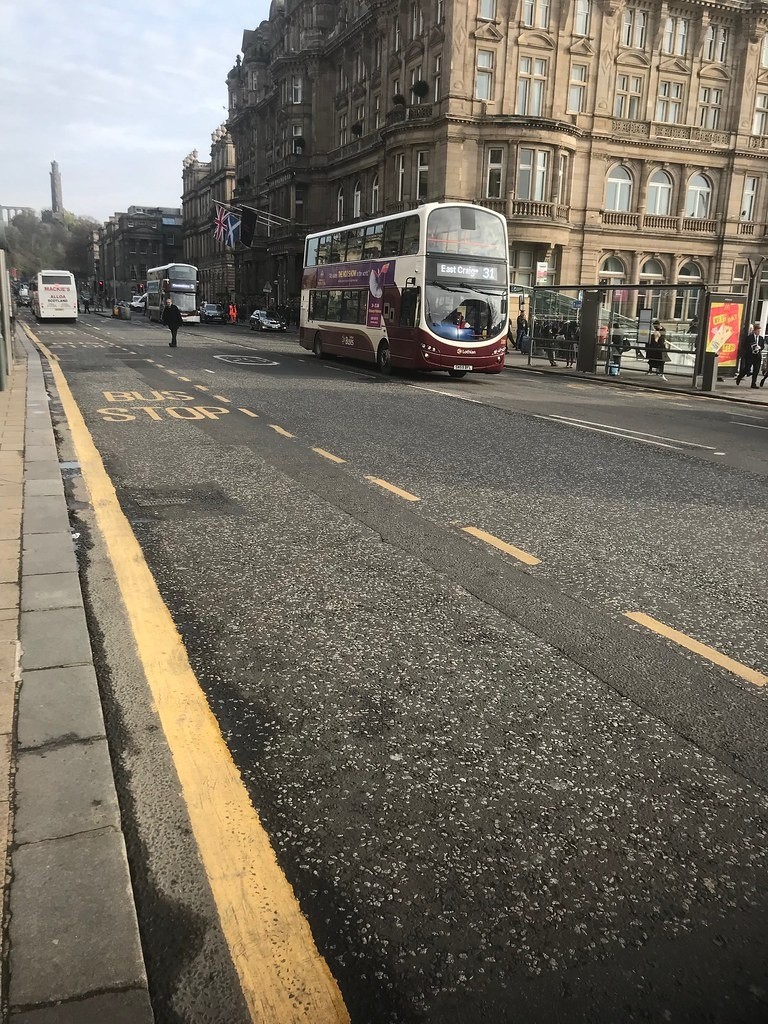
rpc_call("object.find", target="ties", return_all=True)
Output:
[755,336,757,343]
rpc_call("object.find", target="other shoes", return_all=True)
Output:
[751,385,759,388]
[736,377,740,385]
[760,380,764,386]
[89,311,90,314]
[169,343,176,347]
[660,375,667,381]
[645,372,653,375]
[84,312,86,313]
[570,365,573,367]
[566,365,569,367]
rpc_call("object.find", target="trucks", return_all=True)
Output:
[128,292,147,313]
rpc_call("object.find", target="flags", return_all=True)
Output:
[213,205,258,248]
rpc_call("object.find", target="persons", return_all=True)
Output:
[370,247,380,259]
[455,311,466,328]
[687,314,699,335]
[645,322,666,380]
[162,298,183,347]
[384,246,394,258]
[278,298,300,329]
[736,322,768,388]
[219,299,262,325]
[82,295,92,314]
[505,309,580,368]
[605,323,623,375]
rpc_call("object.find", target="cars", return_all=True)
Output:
[199,304,227,324]
[249,310,287,332]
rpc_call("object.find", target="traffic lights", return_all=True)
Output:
[140,284,144,293]
[99,280,104,292]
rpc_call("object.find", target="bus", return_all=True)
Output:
[29,269,78,324]
[145,263,200,326]
[296,202,512,379]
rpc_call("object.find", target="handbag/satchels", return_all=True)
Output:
[623,339,630,351]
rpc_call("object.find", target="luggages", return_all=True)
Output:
[521,336,530,354]
